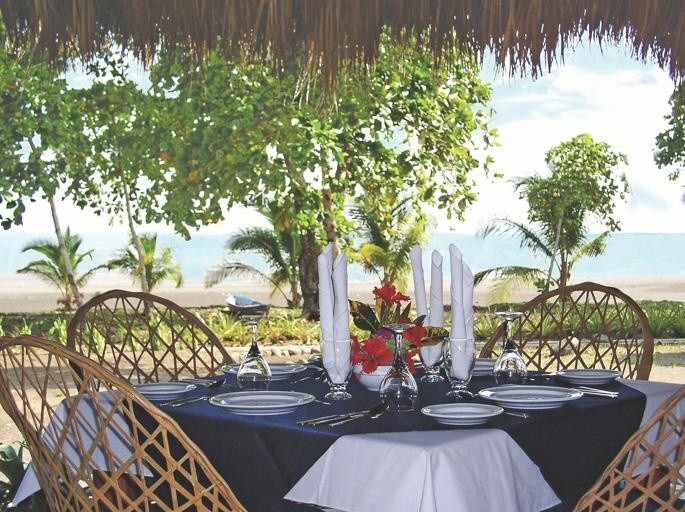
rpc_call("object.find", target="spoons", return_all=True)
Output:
[328,402,389,429]
[172,379,227,389]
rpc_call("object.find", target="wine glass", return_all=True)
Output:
[380,324,416,413]
[236,316,272,390]
[492,311,527,385]
[416,337,477,400]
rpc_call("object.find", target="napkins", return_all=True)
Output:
[447,243,475,381]
[408,243,444,366]
[317,241,353,383]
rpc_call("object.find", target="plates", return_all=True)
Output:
[479,385,564,409]
[129,382,198,400]
[418,402,504,426]
[209,391,317,416]
[221,360,309,381]
[556,370,621,385]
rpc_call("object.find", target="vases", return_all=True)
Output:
[350,363,392,393]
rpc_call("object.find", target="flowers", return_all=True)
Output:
[344,284,446,374]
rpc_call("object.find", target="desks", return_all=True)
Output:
[120,353,685,504]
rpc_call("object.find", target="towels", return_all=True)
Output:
[316,240,475,382]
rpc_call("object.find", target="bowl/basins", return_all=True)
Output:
[353,366,393,390]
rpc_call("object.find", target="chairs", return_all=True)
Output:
[576,383,685,511]
[64,287,235,392]
[479,282,655,381]
[1,331,246,510]
